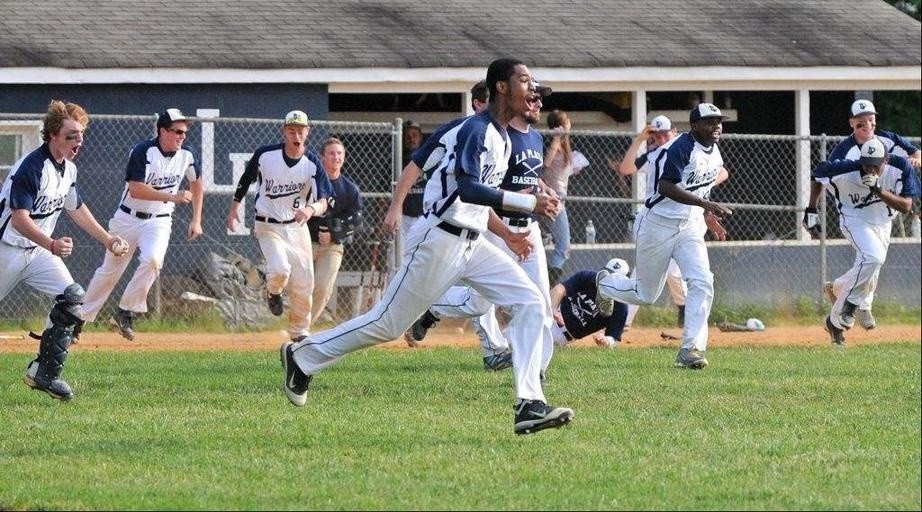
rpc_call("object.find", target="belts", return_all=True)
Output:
[423,211,479,240]
[499,216,536,227]
[557,321,572,341]
[255,215,296,224]
[120,203,169,219]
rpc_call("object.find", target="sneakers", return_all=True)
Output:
[513,399,574,434]
[267,290,283,316]
[859,310,875,328]
[840,298,859,328]
[674,348,708,368]
[292,335,308,341]
[676,305,685,329]
[280,342,313,406]
[109,307,135,341]
[824,315,847,348]
[72,319,85,343]
[404,310,440,346]
[596,270,614,316]
[24,361,74,399]
[823,281,837,305]
[483,350,513,371]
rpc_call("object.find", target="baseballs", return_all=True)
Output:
[112,241,126,255]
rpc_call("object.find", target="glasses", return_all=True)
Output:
[532,95,542,102]
[166,127,189,134]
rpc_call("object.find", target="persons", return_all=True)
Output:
[802,137,920,348]
[404,76,554,381]
[802,100,922,331]
[280,58,574,436]
[69,109,203,346]
[620,115,728,333]
[550,257,631,349]
[0,99,129,405]
[595,103,734,371]
[531,110,573,290]
[377,120,431,261]
[306,139,363,330]
[226,110,330,343]
[382,80,516,373]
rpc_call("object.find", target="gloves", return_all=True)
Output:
[861,173,882,195]
[802,208,822,239]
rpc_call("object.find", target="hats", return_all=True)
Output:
[849,100,878,118]
[157,109,197,127]
[530,76,552,96]
[649,115,676,134]
[403,120,421,131]
[690,104,730,124]
[855,139,888,165]
[284,110,308,128]
[601,258,630,278]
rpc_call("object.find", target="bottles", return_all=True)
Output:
[911,215,921,239]
[586,220,595,244]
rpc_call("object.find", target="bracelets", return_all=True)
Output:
[308,204,318,215]
[319,227,329,232]
[51,239,55,254]
